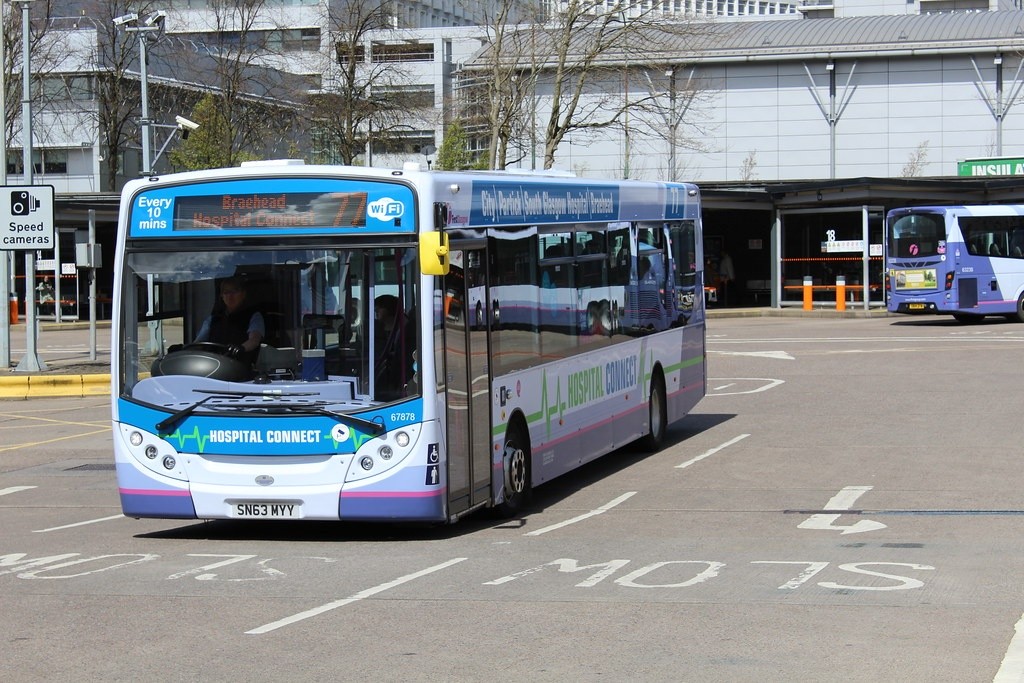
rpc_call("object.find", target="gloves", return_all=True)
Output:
[227,344,245,359]
[168,344,183,354]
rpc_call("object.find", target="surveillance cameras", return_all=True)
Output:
[113,12,139,26]
[145,10,166,27]
[175,115,200,130]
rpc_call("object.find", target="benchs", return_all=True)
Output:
[746,277,823,303]
[35,300,77,323]
[97,298,111,320]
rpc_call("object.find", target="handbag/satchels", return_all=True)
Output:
[375,359,392,379]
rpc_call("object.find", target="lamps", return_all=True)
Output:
[665,68,674,76]
[510,74,517,81]
[993,54,1002,64]
[825,62,834,70]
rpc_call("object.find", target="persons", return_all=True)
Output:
[717,248,738,305]
[169,277,266,361]
[370,296,410,381]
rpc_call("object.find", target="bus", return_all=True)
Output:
[296,231,689,337]
[108,155,709,527]
[883,204,1024,323]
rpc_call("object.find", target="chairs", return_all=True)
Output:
[970,244,1022,257]
[259,312,291,349]
